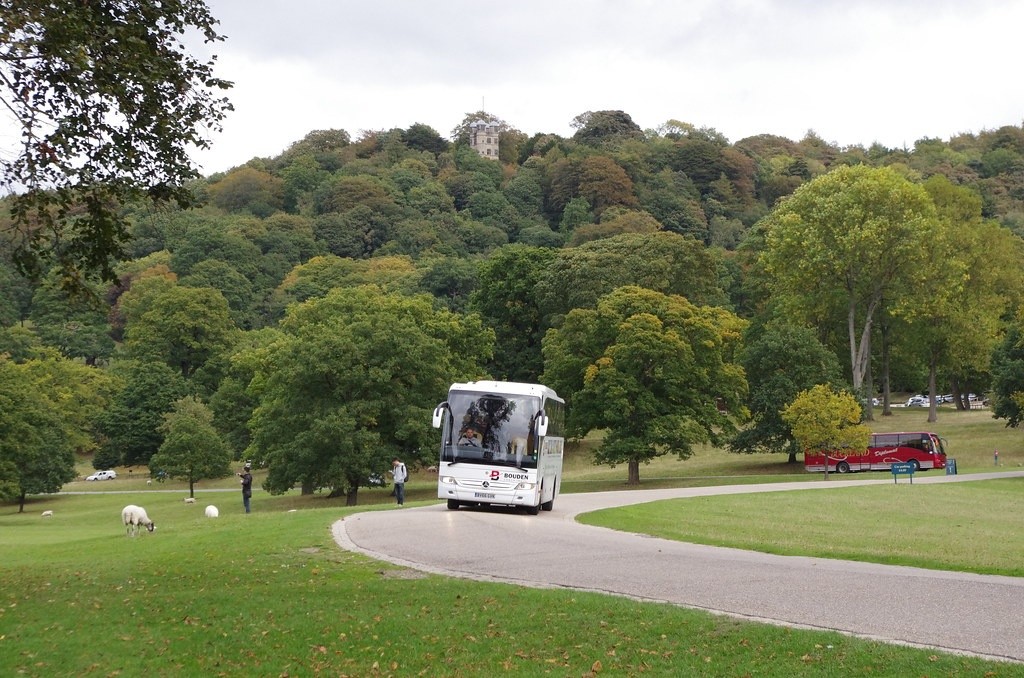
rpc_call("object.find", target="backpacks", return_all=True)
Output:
[395,465,408,482]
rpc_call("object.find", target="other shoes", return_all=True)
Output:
[399,504,404,507]
[394,504,398,507]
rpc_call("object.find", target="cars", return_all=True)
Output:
[367,474,385,488]
[863,392,990,408]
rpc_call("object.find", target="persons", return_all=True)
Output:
[387,458,407,505]
[458,428,483,447]
[237,466,252,512]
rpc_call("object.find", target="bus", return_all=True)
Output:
[432,380,567,515]
[803,430,949,474]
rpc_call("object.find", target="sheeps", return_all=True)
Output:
[205,505,219,518]
[122,505,154,536]
[41,510,53,516]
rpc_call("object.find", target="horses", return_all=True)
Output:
[147,481,151,486]
[184,497,195,503]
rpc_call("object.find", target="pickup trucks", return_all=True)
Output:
[85,470,117,481]
